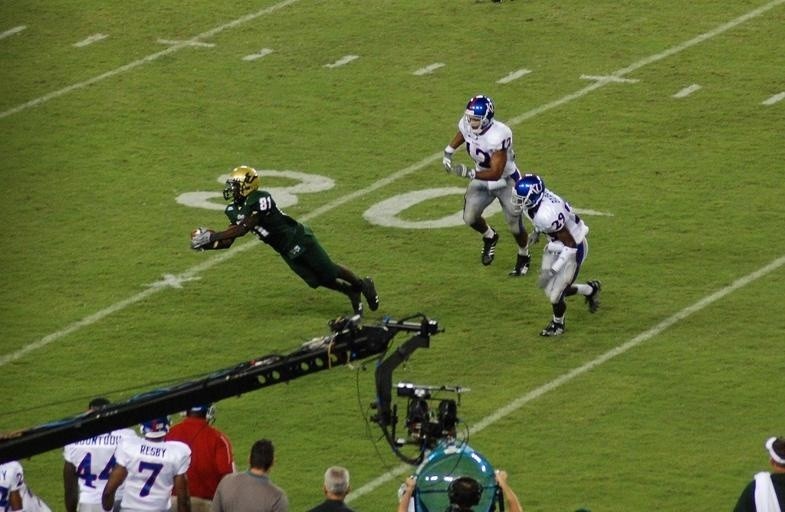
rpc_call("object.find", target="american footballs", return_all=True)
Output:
[191,227,218,252]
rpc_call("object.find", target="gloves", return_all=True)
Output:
[442,145,455,174]
[526,229,540,248]
[453,164,476,180]
[191,228,218,251]
[538,268,556,288]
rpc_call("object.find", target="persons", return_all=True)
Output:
[396,471,524,512]
[306,466,354,512]
[209,439,287,512]
[63,397,137,512]
[167,403,233,512]
[0,461,54,512]
[443,95,530,277]
[509,175,601,338]
[101,414,193,512]
[733,438,785,512]
[189,164,379,316]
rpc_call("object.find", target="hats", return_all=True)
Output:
[766,437,785,465]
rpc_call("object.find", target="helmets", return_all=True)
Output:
[465,95,494,136]
[188,403,215,422]
[448,478,483,506]
[224,166,259,203]
[89,398,112,414]
[511,174,545,216]
[140,416,172,439]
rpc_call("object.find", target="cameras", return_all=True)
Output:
[390,381,473,447]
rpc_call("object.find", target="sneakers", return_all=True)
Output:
[540,318,565,336]
[584,280,601,313]
[481,228,499,265]
[347,286,363,315]
[361,277,379,312]
[508,251,531,278]
